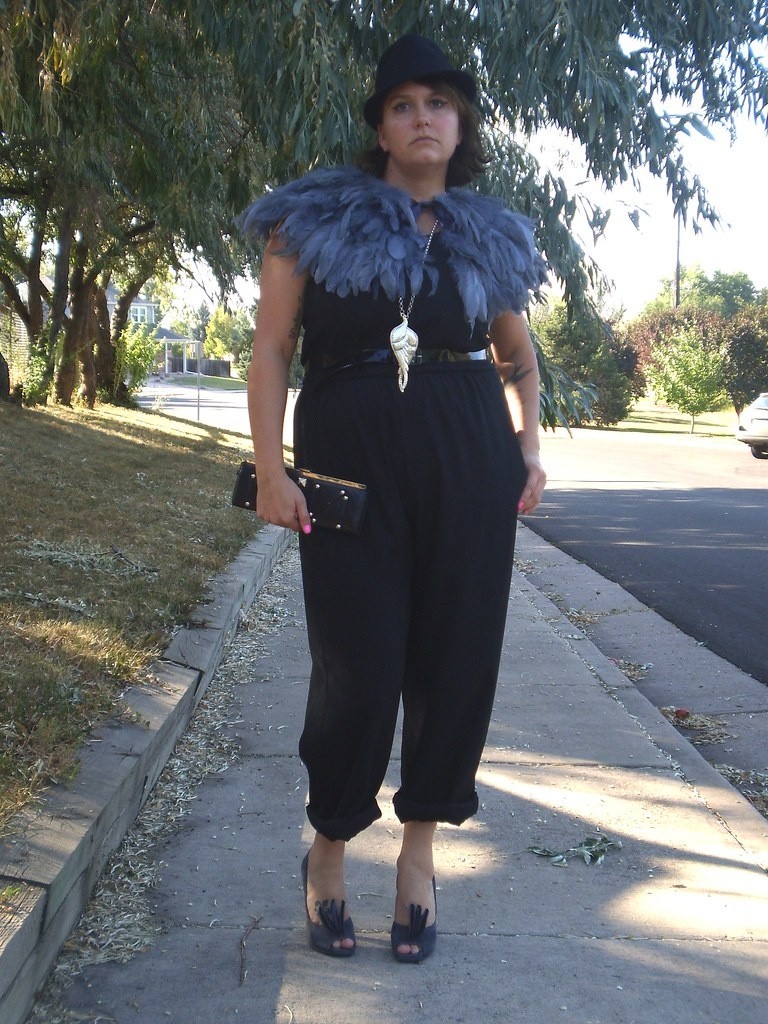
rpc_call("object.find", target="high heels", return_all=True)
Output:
[391,872,437,964]
[301,851,356,956]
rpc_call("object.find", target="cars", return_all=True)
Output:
[736,393,768,459]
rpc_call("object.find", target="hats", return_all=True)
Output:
[363,35,476,131]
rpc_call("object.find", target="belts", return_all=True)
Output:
[304,345,494,380]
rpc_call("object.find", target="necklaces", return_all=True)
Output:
[390,192,447,393]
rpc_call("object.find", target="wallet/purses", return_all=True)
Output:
[232,460,368,538]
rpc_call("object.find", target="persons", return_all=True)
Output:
[246,34,547,965]
